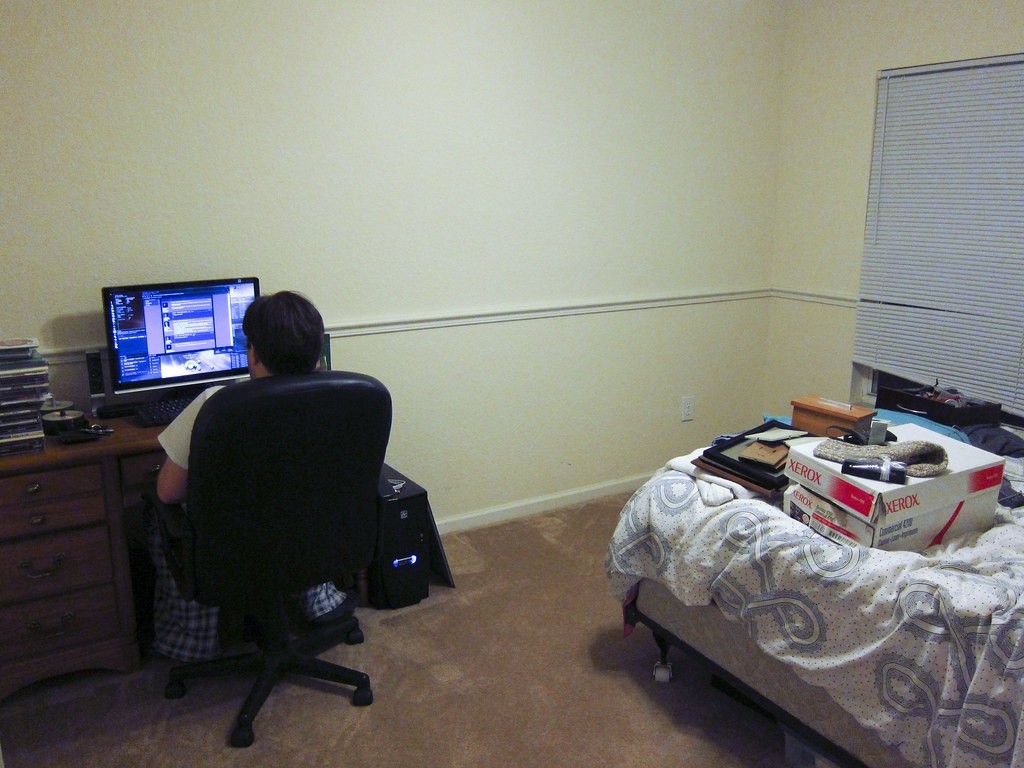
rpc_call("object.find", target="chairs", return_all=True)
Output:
[141,370,394,748]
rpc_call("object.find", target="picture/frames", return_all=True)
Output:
[704,418,819,489]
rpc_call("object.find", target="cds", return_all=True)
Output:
[41,400,86,436]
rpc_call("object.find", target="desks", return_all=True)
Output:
[0,408,371,704]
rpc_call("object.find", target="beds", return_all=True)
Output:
[606,423,1024,768]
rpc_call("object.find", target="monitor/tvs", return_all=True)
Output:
[101,277,261,413]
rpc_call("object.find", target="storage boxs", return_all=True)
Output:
[783,483,1000,554]
[784,423,1006,529]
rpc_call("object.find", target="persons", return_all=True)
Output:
[155,289,325,504]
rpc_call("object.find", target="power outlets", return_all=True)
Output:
[681,396,694,422]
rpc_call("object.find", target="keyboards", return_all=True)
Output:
[134,399,195,428]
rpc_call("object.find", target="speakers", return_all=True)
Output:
[85,348,105,417]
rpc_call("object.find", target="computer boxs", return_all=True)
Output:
[365,461,429,609]
